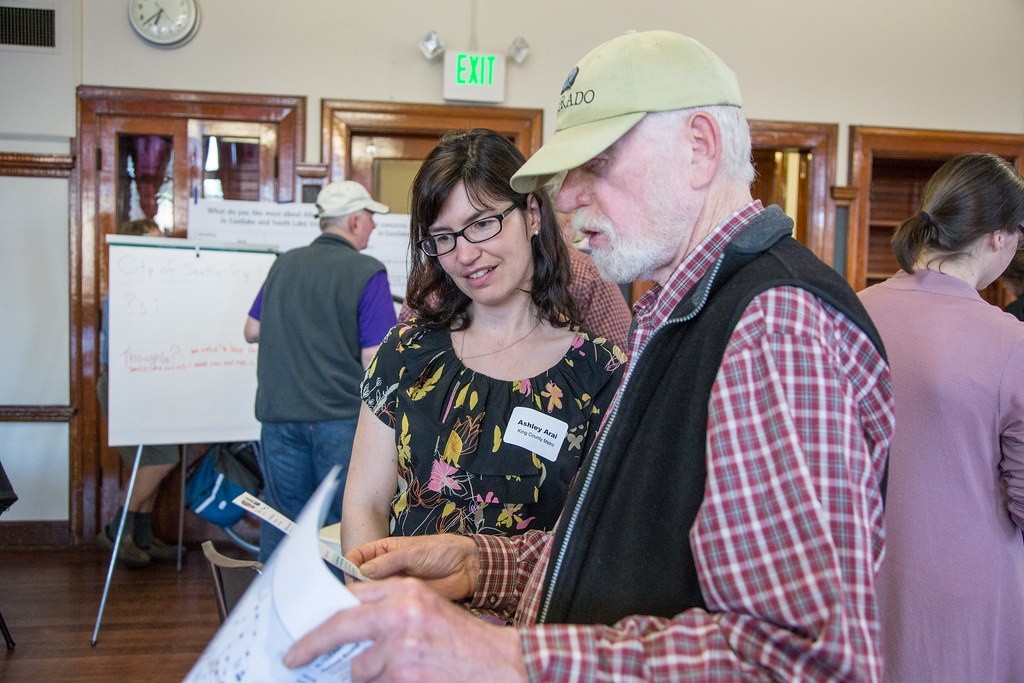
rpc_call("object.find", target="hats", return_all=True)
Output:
[510,29,742,194]
[314,180,389,217]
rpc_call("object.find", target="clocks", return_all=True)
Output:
[128,0,203,50]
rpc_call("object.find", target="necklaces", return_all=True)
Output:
[460,314,545,361]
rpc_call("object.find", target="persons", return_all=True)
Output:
[97,220,186,565]
[244,27,1024,683]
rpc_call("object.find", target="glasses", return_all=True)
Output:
[415,202,519,257]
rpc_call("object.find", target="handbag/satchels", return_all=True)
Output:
[185,444,261,528]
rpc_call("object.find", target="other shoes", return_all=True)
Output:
[95,525,151,565]
[142,538,187,560]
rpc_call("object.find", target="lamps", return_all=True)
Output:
[417,28,445,60]
[506,37,532,64]
